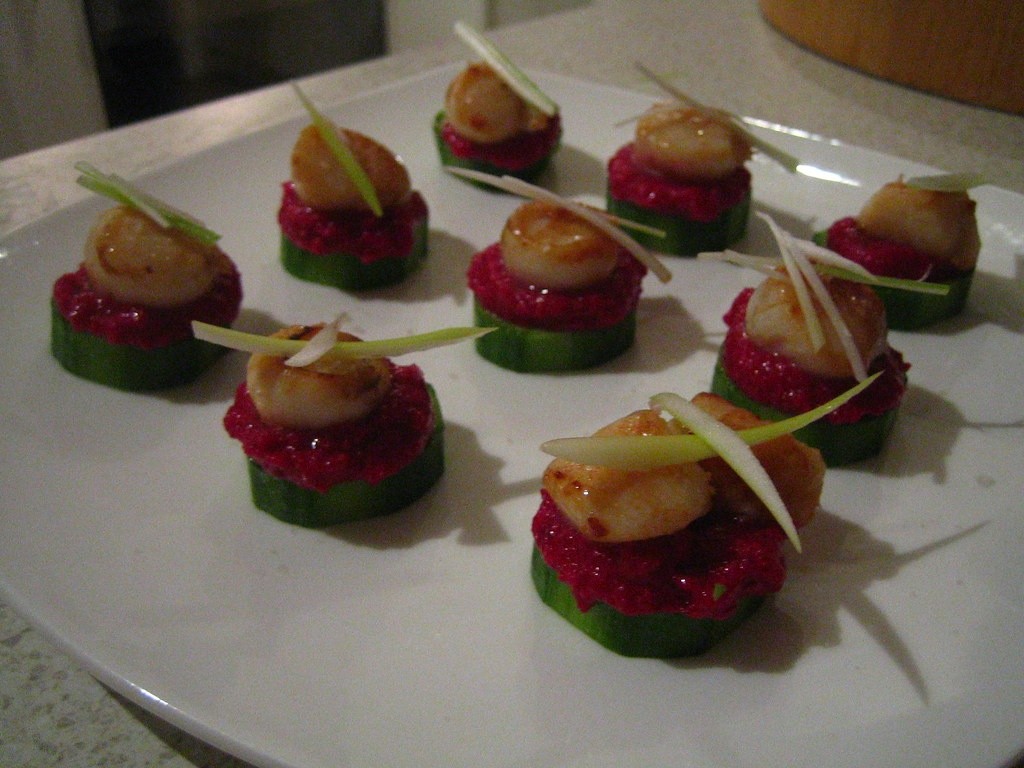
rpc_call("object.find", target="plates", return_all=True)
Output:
[0,5,1024,768]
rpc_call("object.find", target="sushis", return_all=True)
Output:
[51,22,996,661]
